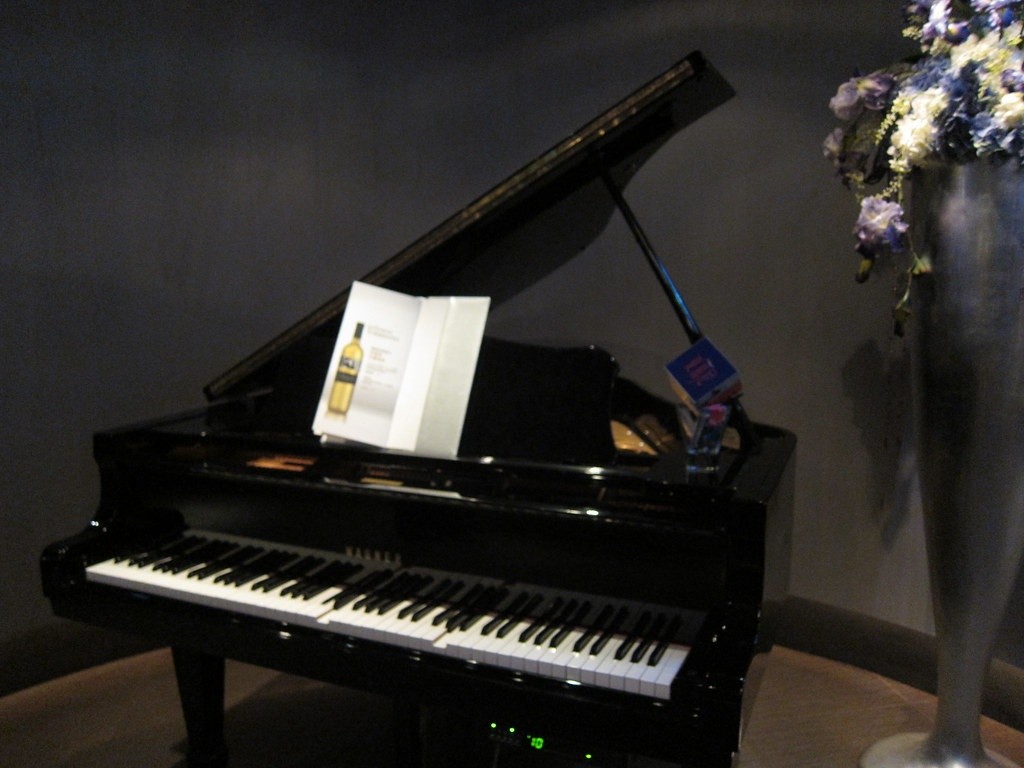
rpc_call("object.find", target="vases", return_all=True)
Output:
[855,157,1023,768]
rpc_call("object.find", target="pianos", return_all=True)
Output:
[40,52,797,768]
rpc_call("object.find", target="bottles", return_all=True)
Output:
[328,323,365,414]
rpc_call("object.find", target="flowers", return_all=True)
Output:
[816,0,1023,338]
